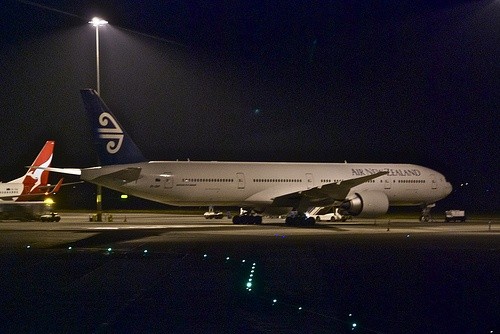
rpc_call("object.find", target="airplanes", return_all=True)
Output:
[26,87,453,224]
[0,139,64,218]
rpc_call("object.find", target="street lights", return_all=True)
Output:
[86,14,108,220]
